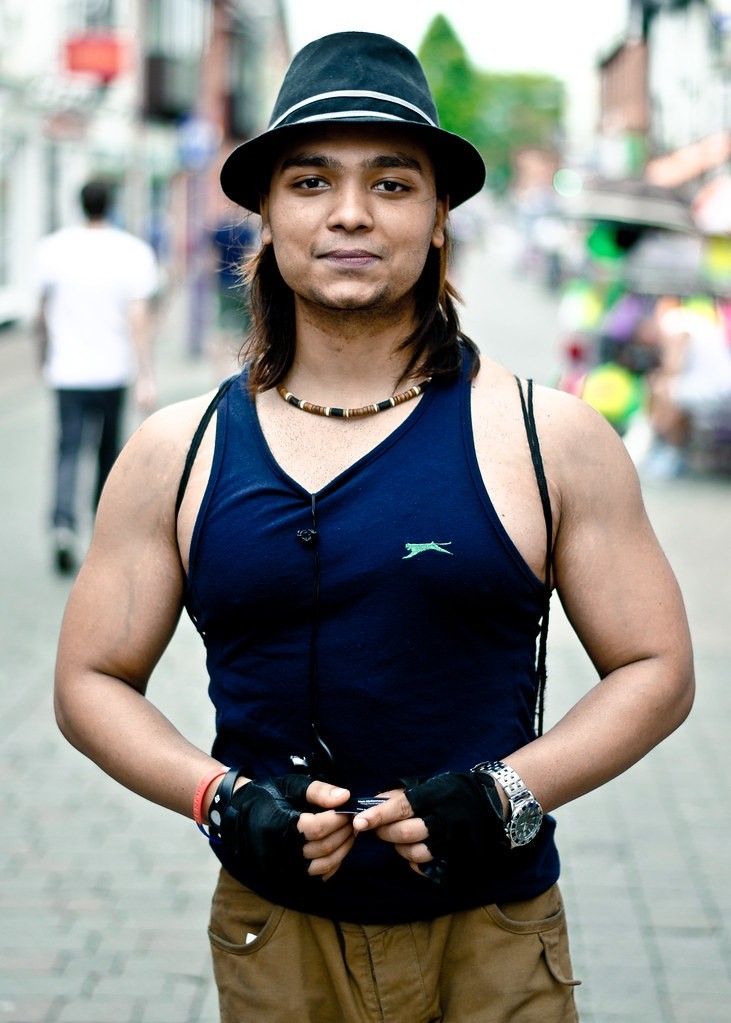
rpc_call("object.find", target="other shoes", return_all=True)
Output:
[55,534,78,571]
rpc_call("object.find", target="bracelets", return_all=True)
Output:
[208,765,249,838]
[193,762,231,837]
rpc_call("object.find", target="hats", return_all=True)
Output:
[221,33,485,210]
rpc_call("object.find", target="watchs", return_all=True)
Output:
[471,759,543,852]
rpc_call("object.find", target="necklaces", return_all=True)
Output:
[274,376,433,419]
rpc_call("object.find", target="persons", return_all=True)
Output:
[30,177,162,575]
[54,30,698,1023]
